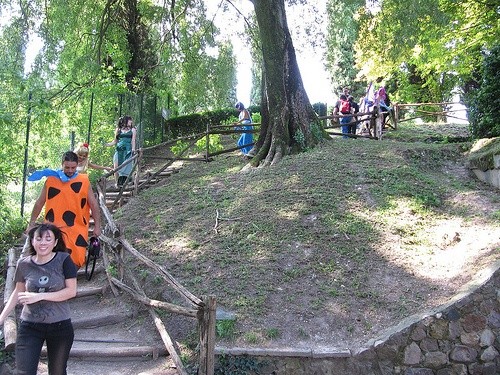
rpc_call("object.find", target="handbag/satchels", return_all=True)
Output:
[89,236,100,255]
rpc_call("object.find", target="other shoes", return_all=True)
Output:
[120,185,123,188]
[115,184,119,190]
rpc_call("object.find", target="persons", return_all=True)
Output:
[333,84,391,139]
[232,102,254,155]
[25,151,102,275]
[0,223,78,375]
[105,115,136,186]
[72,144,112,173]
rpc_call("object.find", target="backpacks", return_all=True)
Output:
[338,99,351,114]
